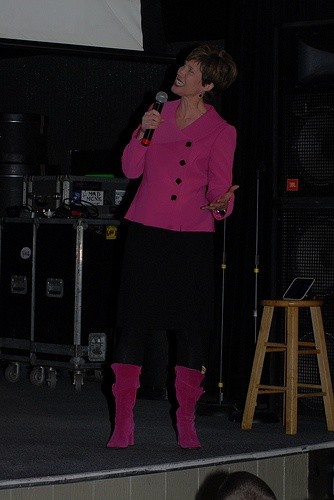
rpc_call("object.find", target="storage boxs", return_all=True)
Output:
[29,175,112,216]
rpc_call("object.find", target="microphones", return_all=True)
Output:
[142,92,169,146]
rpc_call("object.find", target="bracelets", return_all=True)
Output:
[217,209,228,215]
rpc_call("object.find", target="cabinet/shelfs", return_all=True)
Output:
[0,218,122,369]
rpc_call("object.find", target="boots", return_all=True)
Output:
[174,365,204,449]
[107,363,142,449]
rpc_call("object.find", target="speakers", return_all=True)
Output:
[0,111,48,215]
[273,24,334,427]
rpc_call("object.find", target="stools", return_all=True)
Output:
[241,299,334,435]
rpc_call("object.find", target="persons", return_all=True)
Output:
[104,43,239,449]
[195,470,277,500]
